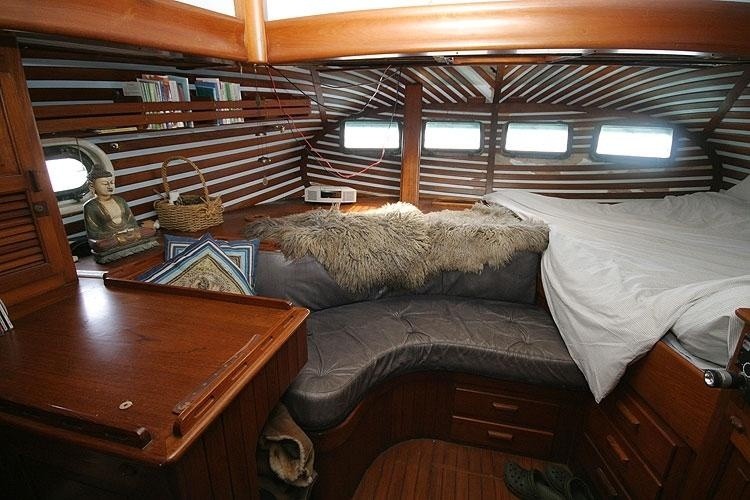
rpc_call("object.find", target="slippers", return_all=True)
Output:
[503,460,565,500]
[547,461,596,500]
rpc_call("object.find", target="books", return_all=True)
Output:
[123,74,244,130]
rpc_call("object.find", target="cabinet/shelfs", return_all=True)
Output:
[450,367,564,458]
[574,381,675,500]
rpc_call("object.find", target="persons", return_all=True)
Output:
[83,165,156,253]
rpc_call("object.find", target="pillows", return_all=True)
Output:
[134,234,261,296]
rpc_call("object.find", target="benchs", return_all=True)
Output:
[264,212,591,430]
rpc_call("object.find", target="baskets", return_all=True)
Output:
[153,156,224,232]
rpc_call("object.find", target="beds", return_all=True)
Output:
[494,176,746,363]
